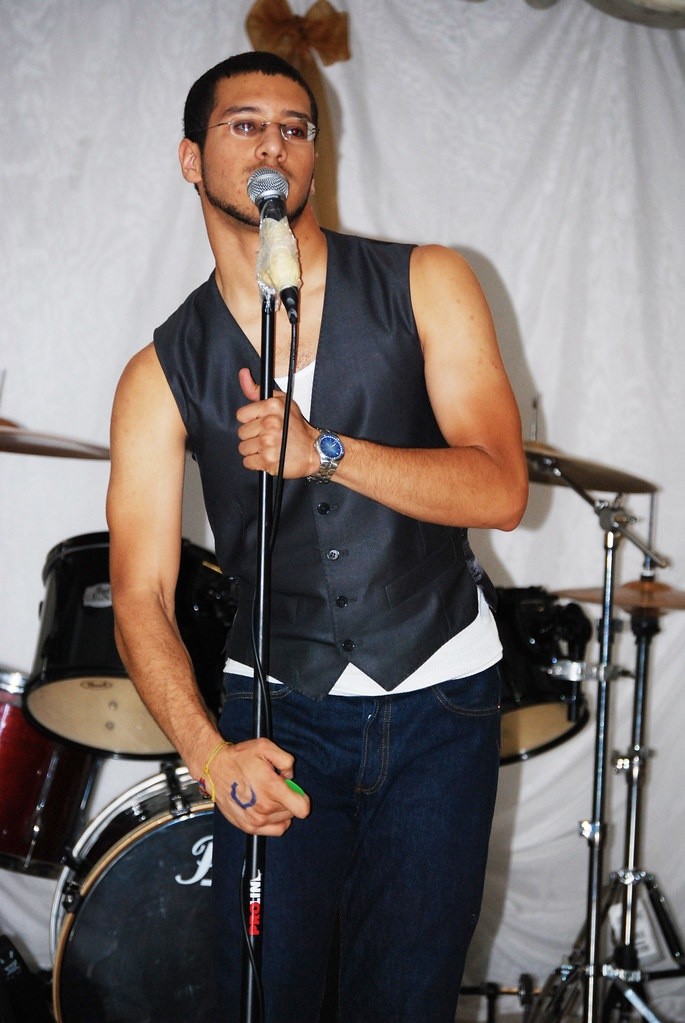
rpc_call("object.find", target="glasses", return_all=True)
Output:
[191,114,320,144]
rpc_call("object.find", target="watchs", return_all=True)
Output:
[309,422,344,490]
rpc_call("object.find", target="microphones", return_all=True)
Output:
[248,166,302,324]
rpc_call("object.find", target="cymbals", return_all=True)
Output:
[550,576,684,610]
[1,416,112,462]
[522,438,661,494]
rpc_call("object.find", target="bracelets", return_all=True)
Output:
[196,741,231,804]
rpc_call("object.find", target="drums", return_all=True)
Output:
[0,665,110,882]
[47,766,263,1023]
[20,527,239,764]
[490,581,590,768]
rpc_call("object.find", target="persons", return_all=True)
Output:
[105,51,529,1023]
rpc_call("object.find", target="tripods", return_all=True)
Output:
[534,468,684,1023]
